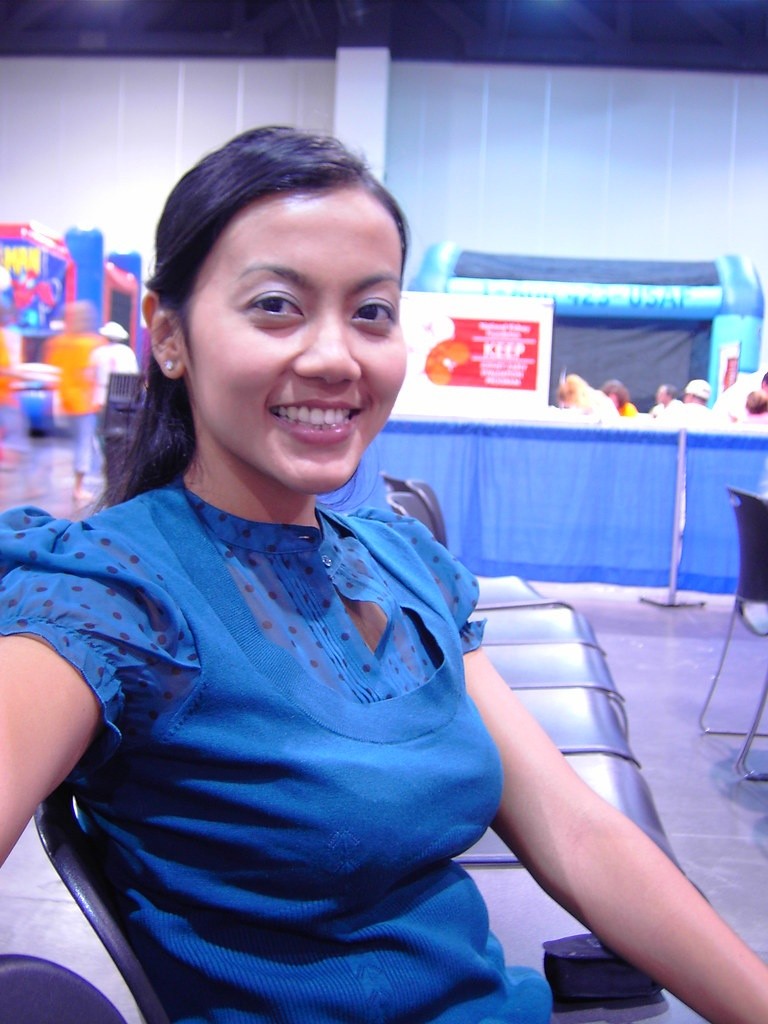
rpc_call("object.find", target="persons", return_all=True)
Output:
[1,126,768,1024]
[88,322,141,409]
[554,369,767,426]
[52,300,100,501]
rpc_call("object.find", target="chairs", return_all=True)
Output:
[0,472,713,1024]
[698,487,767,781]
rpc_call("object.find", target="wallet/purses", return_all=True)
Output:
[542,931,664,998]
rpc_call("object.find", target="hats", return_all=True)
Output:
[685,379,711,402]
[97,321,128,340]
